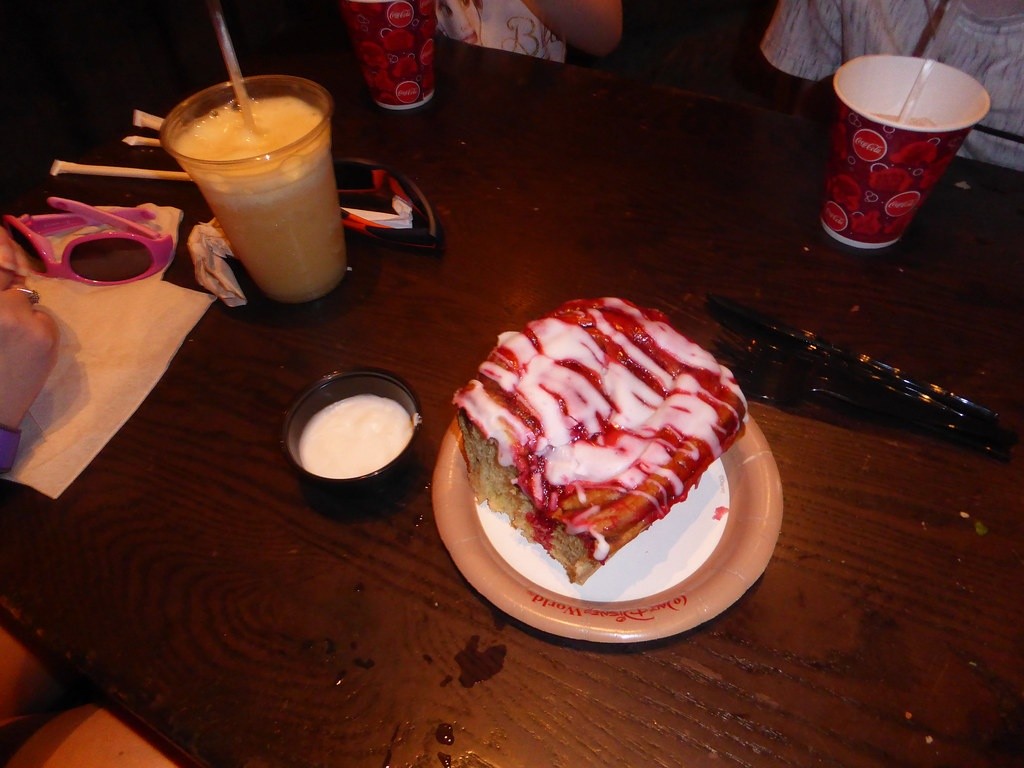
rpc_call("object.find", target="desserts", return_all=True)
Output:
[452,297,747,586]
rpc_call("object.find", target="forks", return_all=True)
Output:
[707,324,1018,462]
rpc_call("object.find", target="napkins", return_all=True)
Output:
[0,279,218,498]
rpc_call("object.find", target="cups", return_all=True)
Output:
[336,0,437,111]
[158,74,350,304]
[279,367,421,521]
[819,55,992,249]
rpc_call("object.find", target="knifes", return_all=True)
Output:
[703,291,1001,427]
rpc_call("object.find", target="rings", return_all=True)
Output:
[13,286,40,305]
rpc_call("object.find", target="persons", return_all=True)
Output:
[438,0,624,64]
[1,223,62,477]
[759,0,1023,171]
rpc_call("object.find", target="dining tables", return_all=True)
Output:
[0,39,1024,768]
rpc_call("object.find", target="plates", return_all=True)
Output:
[431,407,784,644]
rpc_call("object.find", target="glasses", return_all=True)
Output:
[335,157,441,251]
[0,196,174,286]
